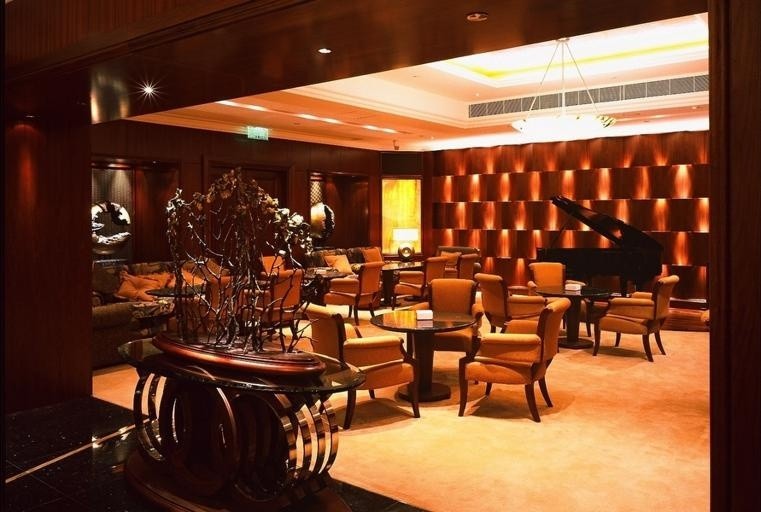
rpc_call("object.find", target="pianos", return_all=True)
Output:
[536,194,663,297]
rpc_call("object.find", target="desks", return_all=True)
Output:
[370,311,476,402]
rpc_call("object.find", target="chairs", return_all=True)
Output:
[593,276,679,362]
[458,297,572,422]
[92,243,612,386]
[299,301,421,429]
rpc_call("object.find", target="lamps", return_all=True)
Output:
[508,35,616,142]
[391,225,423,263]
